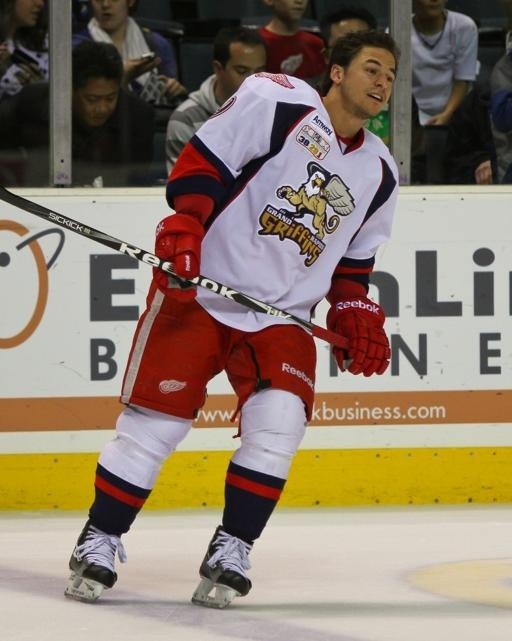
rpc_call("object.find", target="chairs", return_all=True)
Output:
[0,0,512,188]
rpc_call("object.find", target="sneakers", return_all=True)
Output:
[70,519,123,588]
[200,525,254,597]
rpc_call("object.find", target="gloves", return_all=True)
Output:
[153,215,204,302]
[327,297,391,376]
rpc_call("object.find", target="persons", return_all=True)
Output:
[2,1,512,187]
[65,26,401,598]
